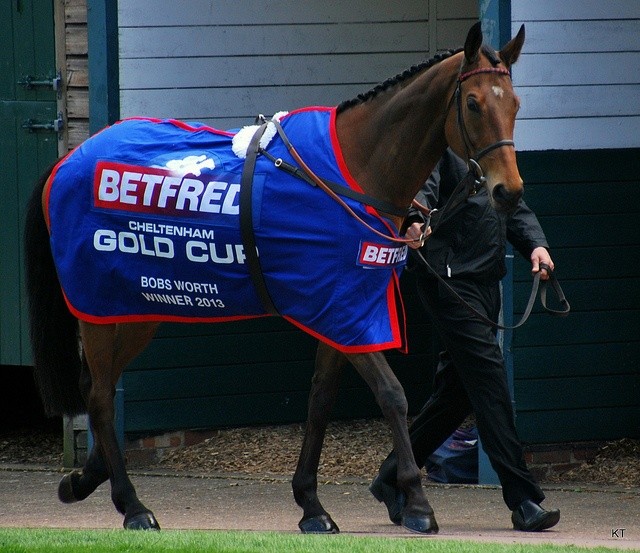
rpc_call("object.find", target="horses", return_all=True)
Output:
[23,20,528,534]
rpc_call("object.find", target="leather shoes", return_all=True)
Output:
[511,498,561,531]
[369,474,402,525]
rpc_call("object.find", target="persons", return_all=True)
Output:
[369,125,560,529]
